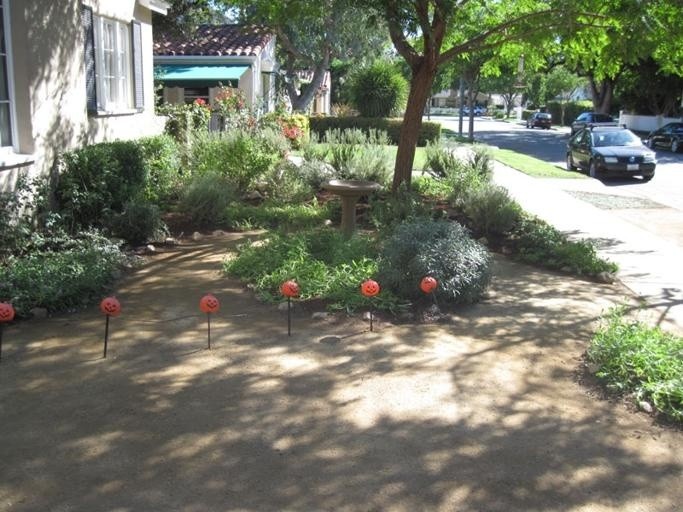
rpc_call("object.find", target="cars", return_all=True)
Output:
[527,113,552,128]
[462,105,487,115]
[648,123,683,152]
[567,128,655,180]
[571,112,613,134]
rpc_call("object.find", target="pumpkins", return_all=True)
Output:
[200,295,219,313]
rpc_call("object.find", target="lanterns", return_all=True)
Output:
[200,293,220,313]
